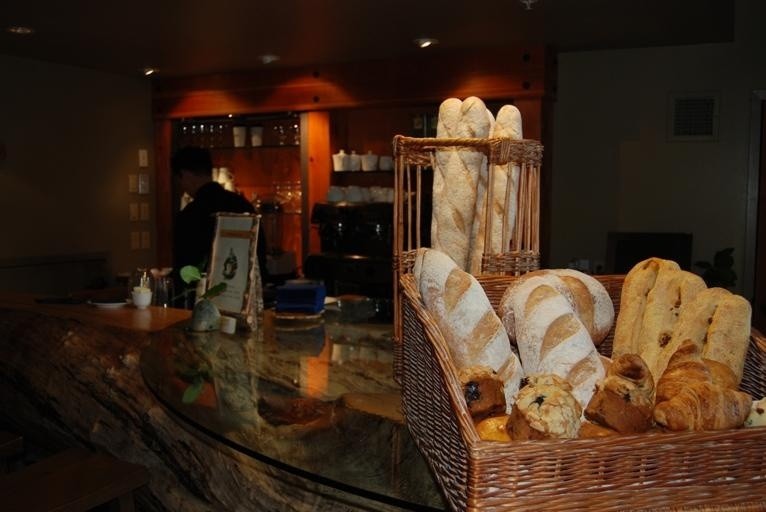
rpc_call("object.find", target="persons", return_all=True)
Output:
[166,143,268,312]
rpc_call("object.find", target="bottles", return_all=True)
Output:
[267,181,301,212]
[179,121,302,145]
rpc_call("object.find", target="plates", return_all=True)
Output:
[88,299,134,310]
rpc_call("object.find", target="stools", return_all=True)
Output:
[1,429,152,512]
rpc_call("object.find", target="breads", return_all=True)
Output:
[454,364,506,423]
[512,276,606,423]
[651,381,752,431]
[518,372,573,393]
[654,288,753,382]
[499,266,616,348]
[430,96,524,274]
[584,353,659,437]
[412,248,528,413]
[637,271,707,385]
[611,255,681,358]
[654,339,713,406]
[563,422,637,488]
[506,384,583,442]
[476,411,512,441]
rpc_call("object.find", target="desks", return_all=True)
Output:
[135,297,454,509]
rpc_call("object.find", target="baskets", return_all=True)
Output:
[400,274,766,512]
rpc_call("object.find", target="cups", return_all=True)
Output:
[327,151,398,204]
[132,289,152,311]
